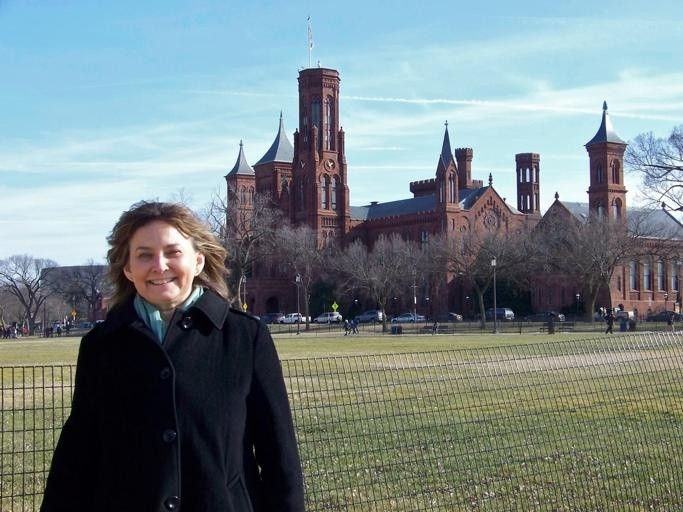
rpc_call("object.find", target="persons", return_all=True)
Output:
[432,320,439,335]
[664,312,674,334]
[343,318,360,335]
[37,198,307,512]
[603,310,615,334]
[0,314,73,341]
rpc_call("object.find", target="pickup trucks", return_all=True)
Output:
[280,312,311,324]
[594,307,634,322]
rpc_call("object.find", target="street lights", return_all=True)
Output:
[489,255,497,333]
[663,293,667,313]
[409,263,418,323]
[295,274,302,336]
[574,293,580,316]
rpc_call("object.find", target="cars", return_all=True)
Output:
[425,312,463,322]
[390,313,424,324]
[79,322,93,329]
[645,310,683,322]
[523,310,565,323]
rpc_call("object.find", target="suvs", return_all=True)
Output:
[260,313,284,323]
[355,310,387,323]
[476,308,515,322]
[312,312,342,324]
